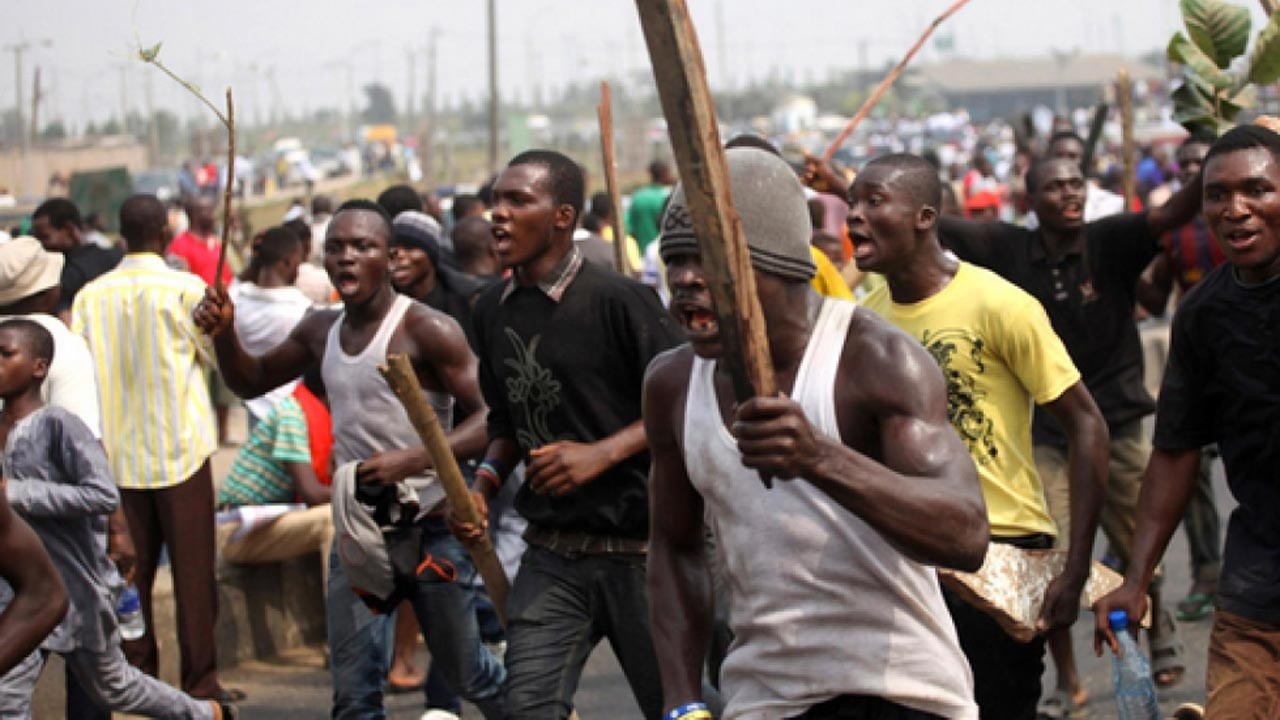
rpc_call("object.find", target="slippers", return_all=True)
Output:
[385,683,426,694]
[1174,587,1215,620]
[1036,689,1094,720]
[1147,610,1187,687]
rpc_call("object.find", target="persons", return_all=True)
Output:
[0,108,1280,720]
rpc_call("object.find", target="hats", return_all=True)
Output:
[391,211,443,266]
[0,235,64,307]
[659,147,817,280]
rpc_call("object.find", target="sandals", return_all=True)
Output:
[215,690,247,703]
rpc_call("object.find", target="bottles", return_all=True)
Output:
[106,578,145,641]
[1107,610,1161,720]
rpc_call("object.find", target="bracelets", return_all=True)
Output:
[475,463,503,488]
[664,701,713,720]
